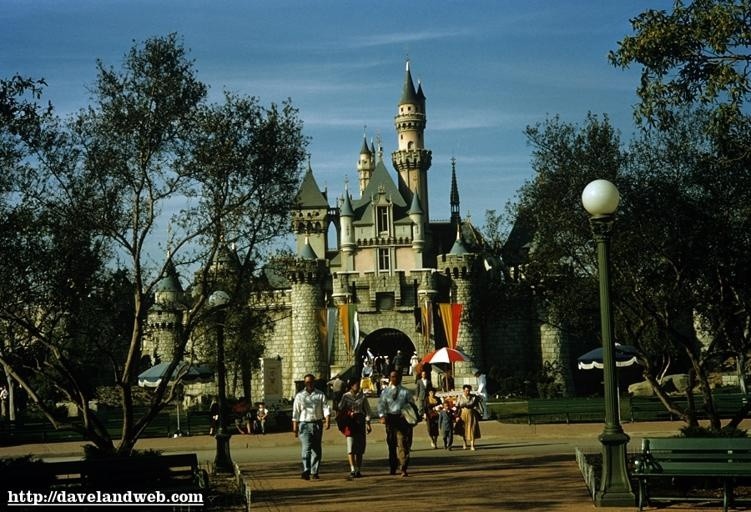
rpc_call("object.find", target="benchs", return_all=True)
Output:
[629,437,750,512]
[0,408,258,445]
[47,454,208,512]
[525,393,750,426]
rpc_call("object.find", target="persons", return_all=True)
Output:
[379,369,417,477]
[293,373,331,482]
[232,395,252,435]
[416,371,433,418]
[208,402,227,436]
[421,386,444,451]
[439,399,454,451]
[325,346,420,417]
[456,383,486,452]
[334,377,373,477]
[255,401,269,433]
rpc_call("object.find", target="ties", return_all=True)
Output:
[392,387,399,401]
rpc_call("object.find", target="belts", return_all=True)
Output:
[304,418,322,424]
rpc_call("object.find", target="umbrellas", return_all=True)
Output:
[139,361,216,429]
[575,345,641,415]
[421,347,469,393]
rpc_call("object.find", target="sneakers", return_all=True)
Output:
[301,472,320,480]
[389,466,409,477]
[431,441,477,451]
[348,471,361,479]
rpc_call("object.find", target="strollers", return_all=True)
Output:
[359,375,376,398]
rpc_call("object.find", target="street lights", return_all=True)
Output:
[199,287,243,479]
[575,174,639,508]
